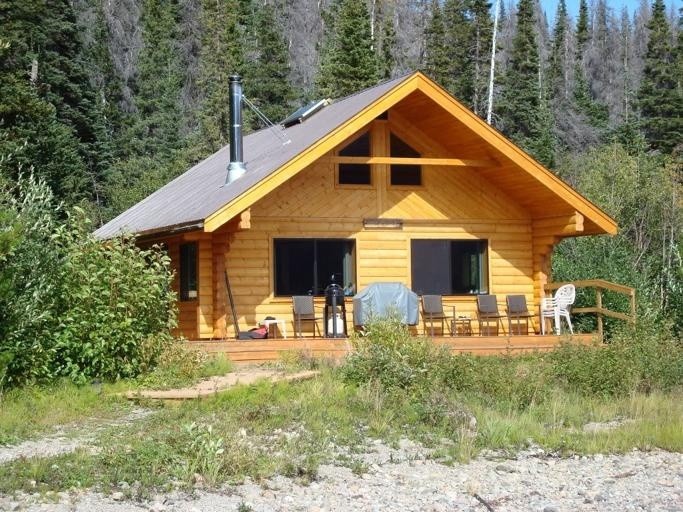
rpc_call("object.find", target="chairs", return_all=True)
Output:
[540,283,576,335]
[505,294,541,334]
[292,294,323,338]
[420,295,457,337]
[476,294,507,336]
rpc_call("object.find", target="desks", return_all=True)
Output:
[259,320,285,340]
[450,317,472,337]
